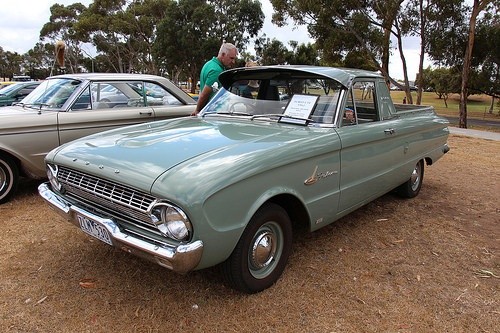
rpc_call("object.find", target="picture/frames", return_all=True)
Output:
[278,93,321,127]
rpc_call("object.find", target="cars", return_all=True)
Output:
[409,86,417,91]
[307,84,321,89]
[93,82,163,107]
[0,83,40,107]
[0,74,197,202]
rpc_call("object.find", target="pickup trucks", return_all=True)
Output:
[38,66,450,295]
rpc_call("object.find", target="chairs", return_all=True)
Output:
[93,97,156,110]
[256,83,281,114]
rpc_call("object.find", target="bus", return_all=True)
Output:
[13,76,31,81]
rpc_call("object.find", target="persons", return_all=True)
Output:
[240,60,260,114]
[190,43,237,116]
